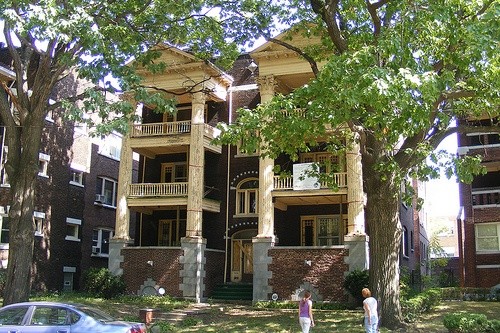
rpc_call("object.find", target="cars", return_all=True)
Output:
[0,301,149,333]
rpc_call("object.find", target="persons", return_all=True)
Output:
[299,290,314,333]
[362,288,378,333]
